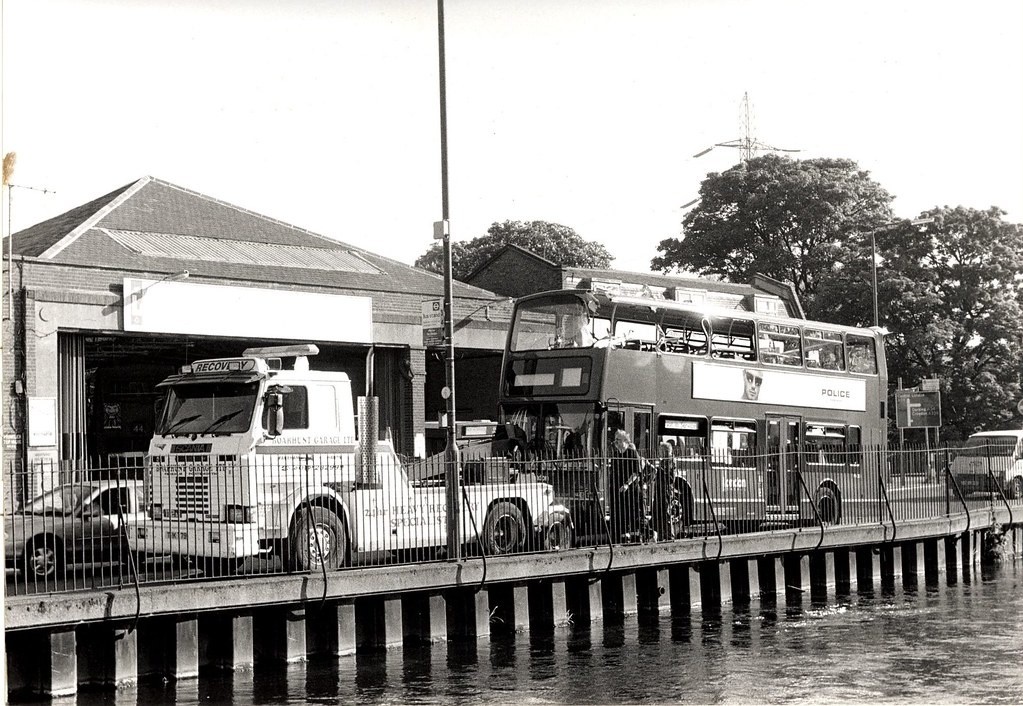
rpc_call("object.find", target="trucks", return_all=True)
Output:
[948,430,1023,499]
[129,343,570,575]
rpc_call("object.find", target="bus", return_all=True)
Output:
[492,289,888,537]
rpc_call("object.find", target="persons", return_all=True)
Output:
[608,431,676,544]
[82,502,104,516]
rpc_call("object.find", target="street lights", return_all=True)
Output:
[872,218,933,326]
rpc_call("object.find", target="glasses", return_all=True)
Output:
[745,371,762,386]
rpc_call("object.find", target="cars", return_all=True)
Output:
[4,480,146,580]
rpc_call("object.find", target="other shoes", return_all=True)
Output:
[646,530,658,542]
[620,532,631,543]
